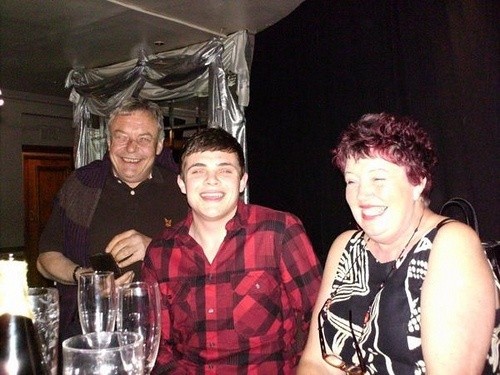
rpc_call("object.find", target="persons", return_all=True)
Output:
[295,113,500,375]
[138,127,323,375]
[36,103,188,375]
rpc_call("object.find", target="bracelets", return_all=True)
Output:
[72,267,84,284]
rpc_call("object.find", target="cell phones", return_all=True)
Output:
[89,253,122,278]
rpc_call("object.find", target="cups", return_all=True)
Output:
[115,282,161,375]
[62,331,146,375]
[28,287,60,375]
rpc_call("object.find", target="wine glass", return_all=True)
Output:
[77,271,117,375]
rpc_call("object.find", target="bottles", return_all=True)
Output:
[0,247,52,375]
